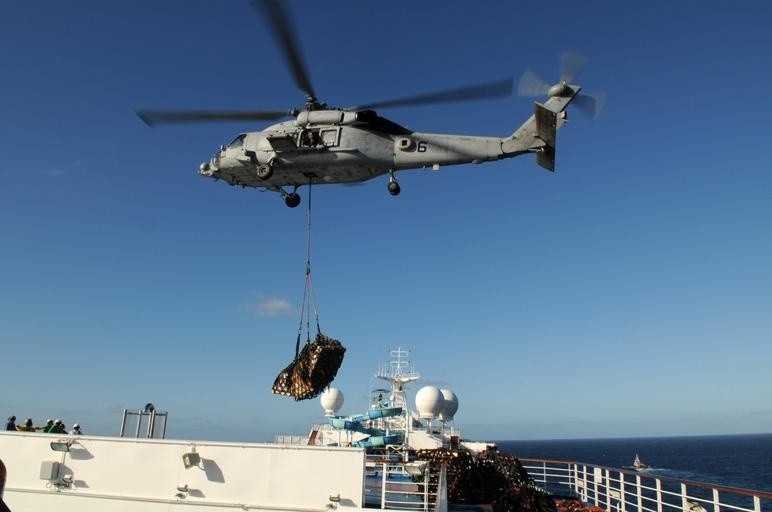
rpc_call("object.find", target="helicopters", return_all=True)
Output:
[135,0,606,208]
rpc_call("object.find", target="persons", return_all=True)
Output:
[4,416,81,434]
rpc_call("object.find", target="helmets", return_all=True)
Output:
[47,420,80,430]
[8,416,16,421]
[25,419,32,426]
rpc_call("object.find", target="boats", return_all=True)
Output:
[633,453,649,468]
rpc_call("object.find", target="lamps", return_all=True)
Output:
[183,444,201,469]
[51,439,74,452]
